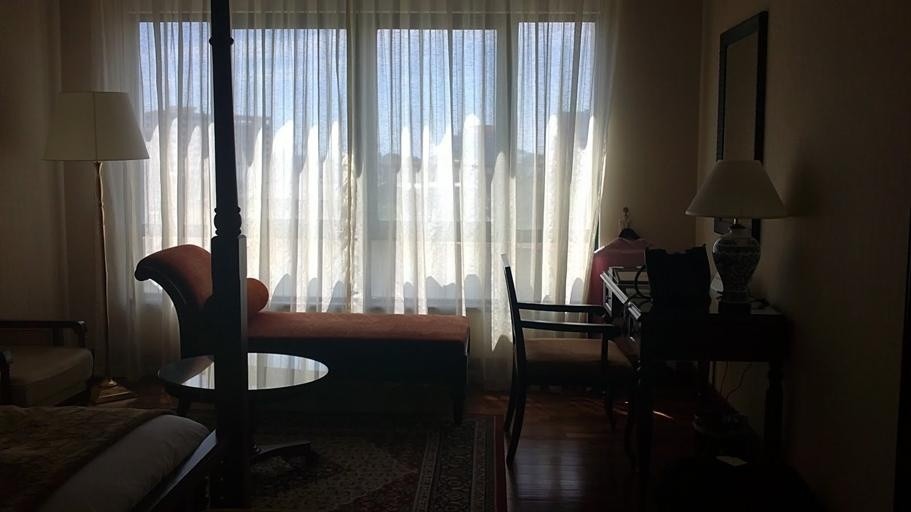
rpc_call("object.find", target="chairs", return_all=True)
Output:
[1,316,97,406]
[131,241,474,427]
[499,250,633,470]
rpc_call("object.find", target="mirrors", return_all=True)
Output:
[713,10,771,247]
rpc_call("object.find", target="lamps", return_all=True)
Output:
[683,158,794,305]
[41,88,151,406]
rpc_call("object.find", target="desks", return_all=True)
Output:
[598,267,793,470]
[157,350,331,462]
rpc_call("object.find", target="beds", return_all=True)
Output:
[0,401,221,512]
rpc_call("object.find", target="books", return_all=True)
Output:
[607,266,649,283]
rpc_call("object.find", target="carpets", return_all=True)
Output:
[167,407,510,510]
